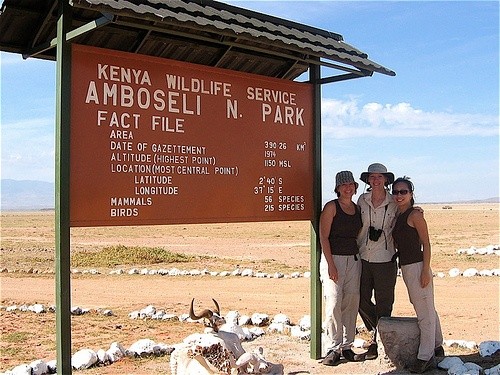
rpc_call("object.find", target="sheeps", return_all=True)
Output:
[168,294,275,375]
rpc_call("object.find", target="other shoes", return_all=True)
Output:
[409,356,434,373]
[324,350,340,365]
[342,349,360,361]
[435,347,444,356]
[365,343,378,359]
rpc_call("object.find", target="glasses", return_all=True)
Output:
[392,190,410,195]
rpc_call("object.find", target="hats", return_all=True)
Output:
[360,163,394,186]
[335,171,358,192]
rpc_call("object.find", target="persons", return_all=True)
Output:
[387,177,444,372]
[319,171,363,366]
[355,163,423,361]
